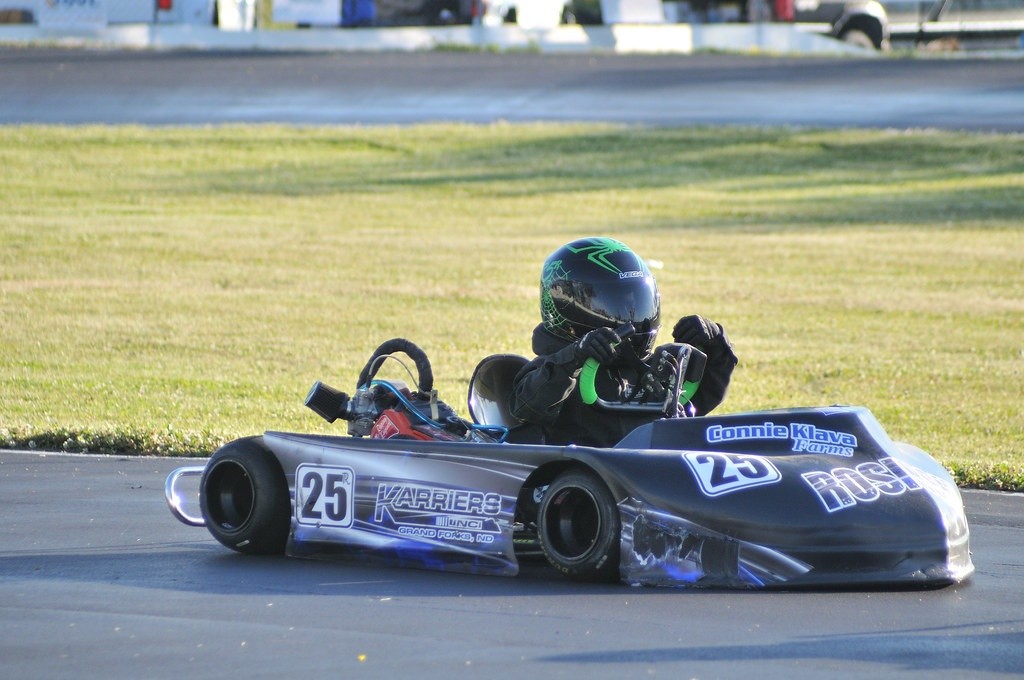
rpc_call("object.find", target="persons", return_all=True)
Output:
[833,0,888,51]
[511,236,738,450]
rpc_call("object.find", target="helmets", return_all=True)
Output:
[540,237,661,364]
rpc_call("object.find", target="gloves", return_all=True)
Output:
[577,326,622,366]
[671,315,723,348]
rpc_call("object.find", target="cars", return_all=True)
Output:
[0,0,1024,56]
